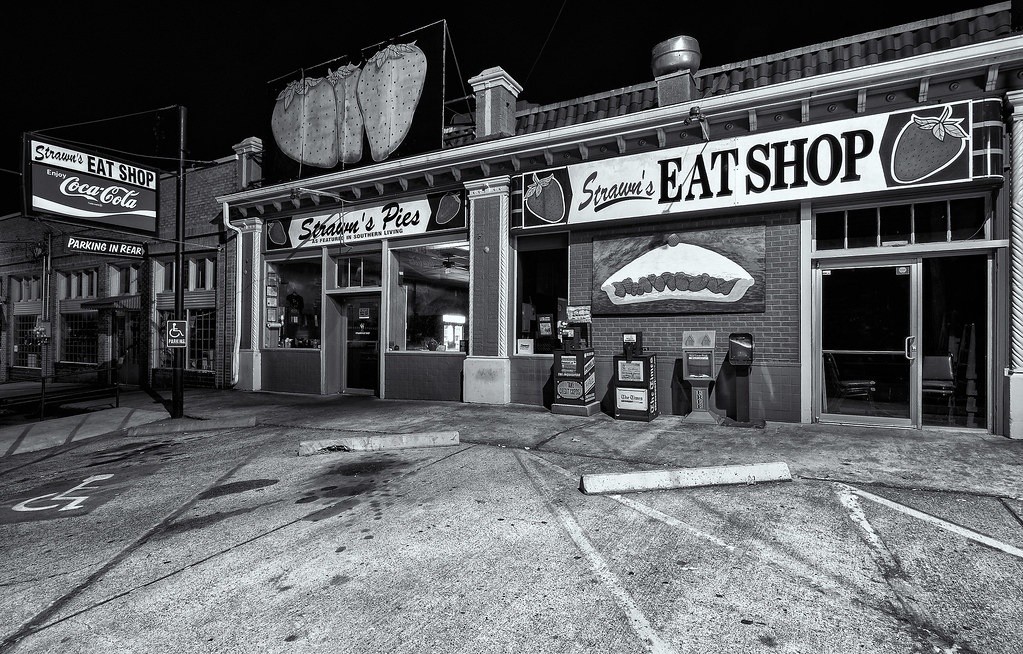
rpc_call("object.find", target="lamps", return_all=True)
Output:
[441,254,456,268]
[683,104,705,127]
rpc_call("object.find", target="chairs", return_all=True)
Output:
[826,352,877,416]
[921,351,958,425]
